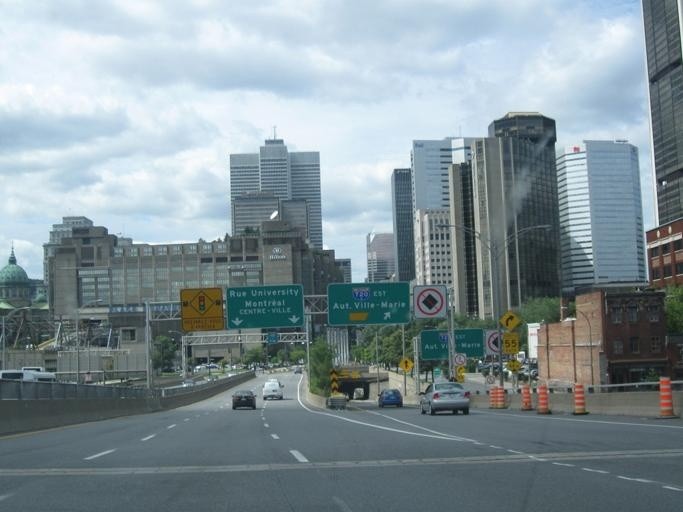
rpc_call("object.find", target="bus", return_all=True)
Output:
[1,366,56,383]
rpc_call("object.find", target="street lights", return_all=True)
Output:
[429,217,554,399]
[1,304,30,379]
[540,317,551,385]
[560,304,595,392]
[74,297,105,386]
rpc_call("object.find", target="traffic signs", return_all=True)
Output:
[501,333,519,354]
[327,280,413,328]
[266,332,279,344]
[225,284,307,329]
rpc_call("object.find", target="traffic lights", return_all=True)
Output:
[419,327,487,360]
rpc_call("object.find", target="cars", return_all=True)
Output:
[468,356,539,383]
[228,371,238,379]
[294,366,305,375]
[417,381,472,418]
[160,361,288,372]
[229,388,257,412]
[182,379,195,388]
[205,375,221,382]
[260,378,284,399]
[375,386,405,407]
